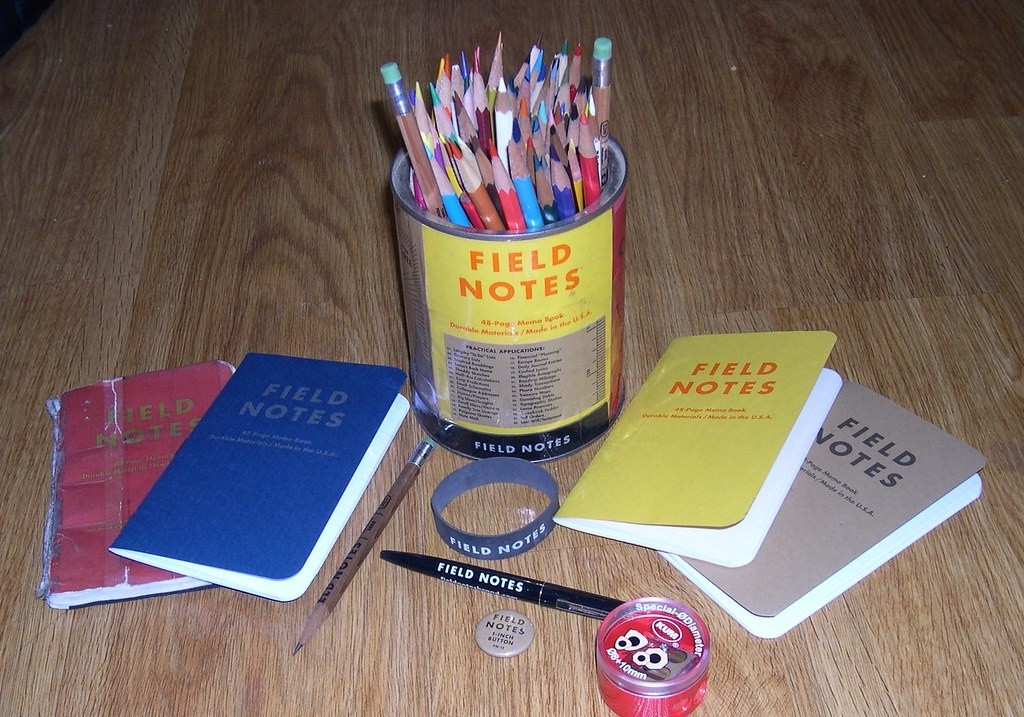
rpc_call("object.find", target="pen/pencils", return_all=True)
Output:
[590,36,617,182]
[409,28,601,231]
[379,545,637,626]
[284,433,435,656]
[378,60,447,220]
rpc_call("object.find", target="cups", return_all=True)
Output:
[386,138,627,461]
